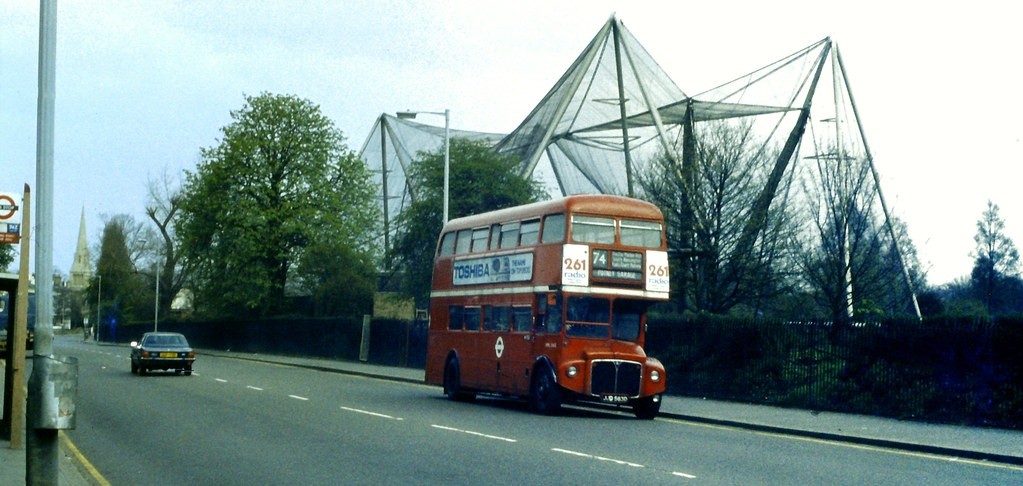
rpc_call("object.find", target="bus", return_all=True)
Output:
[422,193,672,421]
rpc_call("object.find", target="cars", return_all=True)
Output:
[130,334,196,376]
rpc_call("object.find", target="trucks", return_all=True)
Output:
[0,290,36,354]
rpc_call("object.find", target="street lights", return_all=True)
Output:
[54,286,64,335]
[84,275,102,342]
[397,111,449,227]
[138,238,160,332]
